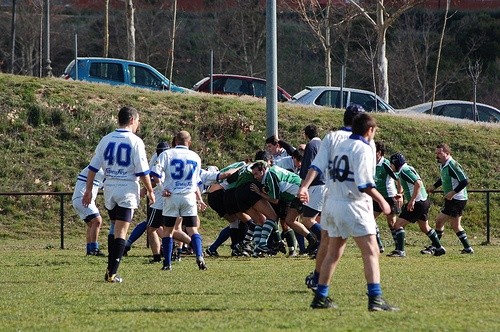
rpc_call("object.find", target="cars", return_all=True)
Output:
[61,58,192,93]
[190,73,292,103]
[398,99,500,125]
[289,84,394,114]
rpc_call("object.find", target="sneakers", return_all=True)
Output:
[460,248,474,255]
[389,249,406,257]
[380,249,398,256]
[432,247,446,256]
[422,245,436,255]
[160,265,172,272]
[86,248,106,257]
[367,296,390,312]
[197,260,208,271]
[104,274,123,283]
[306,274,319,293]
[311,295,338,308]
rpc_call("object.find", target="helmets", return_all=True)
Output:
[390,154,405,172]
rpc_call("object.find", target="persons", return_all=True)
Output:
[72,161,106,257]
[385,152,446,257]
[420,141,475,256]
[309,112,401,313]
[296,103,377,295]
[82,106,156,285]
[121,124,328,263]
[373,139,399,254]
[148,131,208,273]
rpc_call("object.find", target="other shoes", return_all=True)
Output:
[107,246,317,264]
